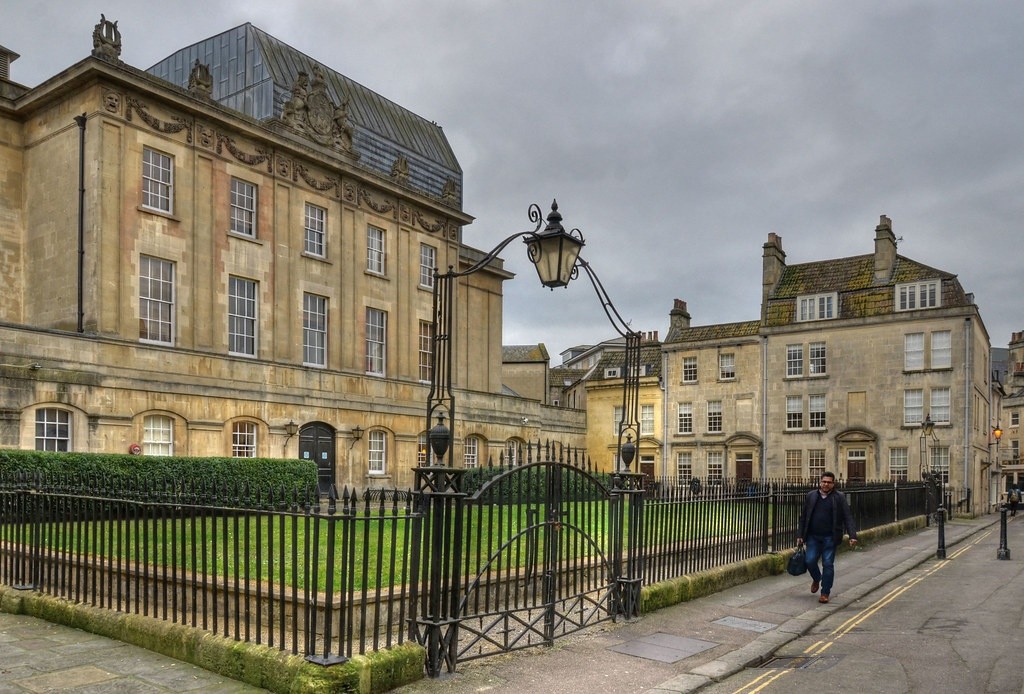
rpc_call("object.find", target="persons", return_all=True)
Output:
[1007,484,1022,516]
[796,471,859,604]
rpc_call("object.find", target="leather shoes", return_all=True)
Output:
[819,594,828,603]
[811,577,822,593]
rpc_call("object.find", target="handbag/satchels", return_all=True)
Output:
[787,543,807,576]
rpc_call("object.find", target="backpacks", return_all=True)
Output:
[1011,490,1019,502]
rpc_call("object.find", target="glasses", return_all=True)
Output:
[821,481,834,484]
